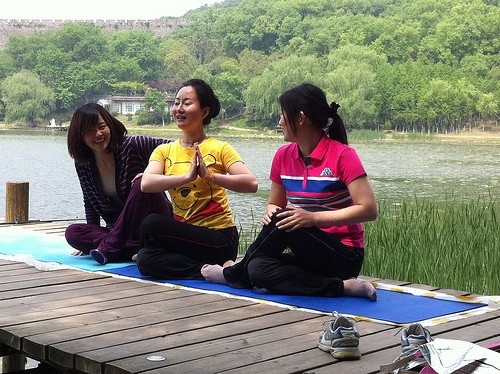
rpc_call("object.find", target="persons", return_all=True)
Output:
[201,84,378,303]
[132,79,258,281]
[65,103,176,265]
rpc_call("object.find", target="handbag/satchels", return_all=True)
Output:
[379,335,500,374]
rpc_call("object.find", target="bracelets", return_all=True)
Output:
[203,169,215,184]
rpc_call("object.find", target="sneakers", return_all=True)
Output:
[317,311,362,360]
[394,322,433,359]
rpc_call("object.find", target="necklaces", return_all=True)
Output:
[181,139,194,143]
